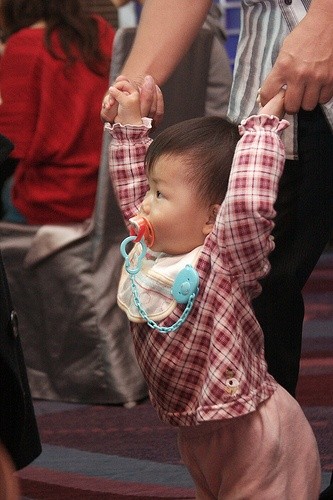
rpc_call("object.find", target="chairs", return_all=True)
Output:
[0,27,214,409]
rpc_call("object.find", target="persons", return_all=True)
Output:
[101,0,333,500]
[0,0,116,224]
[102,75,321,500]
